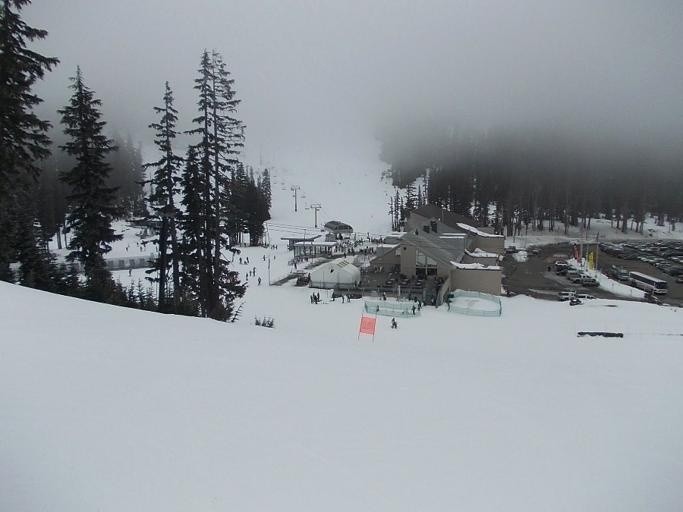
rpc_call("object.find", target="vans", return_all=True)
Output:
[553,261,577,279]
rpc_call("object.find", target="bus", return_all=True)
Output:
[628,269,669,296]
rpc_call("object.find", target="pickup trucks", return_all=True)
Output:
[573,274,591,284]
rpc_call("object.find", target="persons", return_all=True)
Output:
[125,228,435,329]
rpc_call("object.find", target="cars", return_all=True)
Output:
[583,277,602,288]
[559,290,602,301]
[600,238,683,283]
